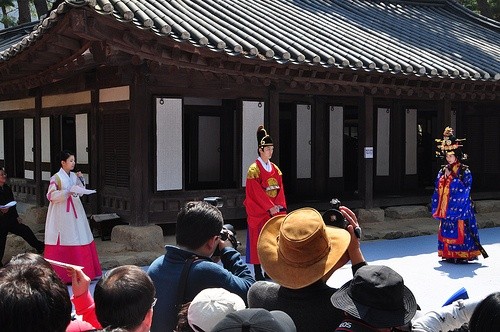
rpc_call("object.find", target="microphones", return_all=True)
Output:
[76,169,85,186]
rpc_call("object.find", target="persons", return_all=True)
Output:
[5,252,102,332]
[0,263,72,332]
[80,264,156,332]
[431,126,489,264]
[0,167,45,267]
[211,307,296,332]
[329,264,483,332]
[247,205,367,332]
[148,201,256,332]
[469,291,500,332]
[43,150,102,284]
[173,287,246,332]
[243,125,287,281]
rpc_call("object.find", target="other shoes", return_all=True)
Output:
[37,241,45,253]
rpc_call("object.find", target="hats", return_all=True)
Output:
[188,288,245,332]
[256,125,274,146]
[257,207,350,289]
[211,308,297,332]
[331,265,417,328]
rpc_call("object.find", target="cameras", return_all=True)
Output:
[322,200,350,230]
[214,224,237,256]
